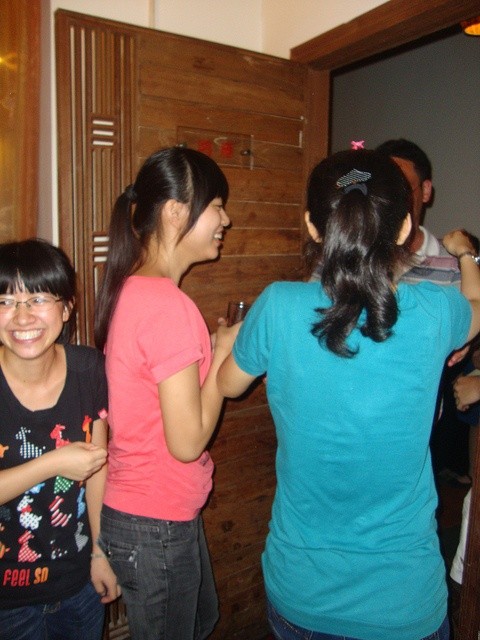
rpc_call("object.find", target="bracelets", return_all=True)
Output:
[88,553,107,559]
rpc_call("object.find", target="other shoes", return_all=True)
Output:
[442,470,471,487]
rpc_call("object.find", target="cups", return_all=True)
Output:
[226,301,251,328]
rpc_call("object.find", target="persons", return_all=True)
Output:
[307,139,472,369]
[91,141,246,640]
[0,237,122,640]
[431,335,480,586]
[215,138,480,640]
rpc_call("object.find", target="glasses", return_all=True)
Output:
[0,297,64,308]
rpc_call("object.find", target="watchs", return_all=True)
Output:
[458,250,480,267]
